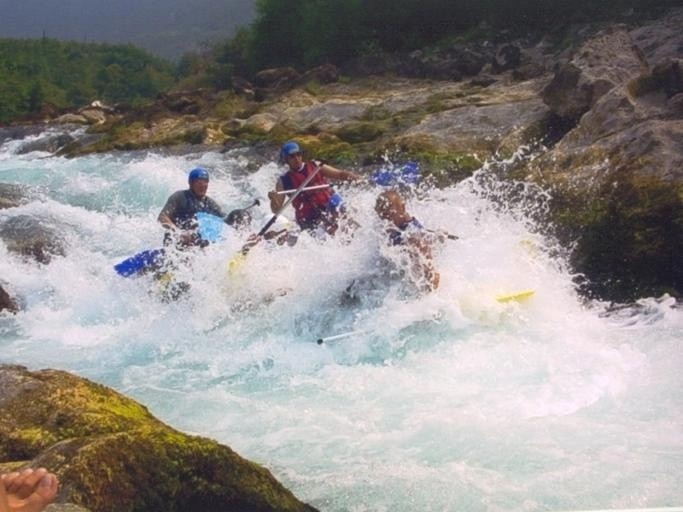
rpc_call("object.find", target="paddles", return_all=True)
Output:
[277,161,423,196]
[113,199,260,279]
[318,290,534,345]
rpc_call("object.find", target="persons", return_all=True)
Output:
[339,191,439,309]
[0,465,58,512]
[267,143,361,237]
[157,170,226,248]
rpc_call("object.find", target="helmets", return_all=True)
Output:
[189,169,209,185]
[375,191,404,210]
[282,142,304,160]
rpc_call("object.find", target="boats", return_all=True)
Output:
[136,232,547,349]
